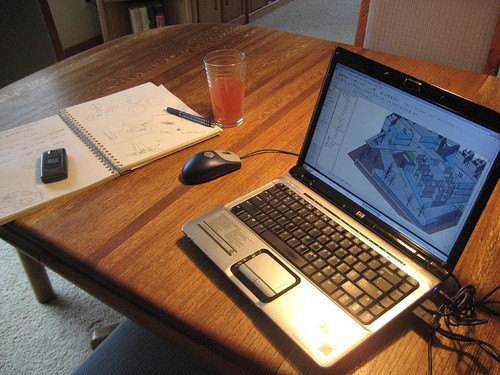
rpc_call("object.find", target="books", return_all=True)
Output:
[0,81,224,227]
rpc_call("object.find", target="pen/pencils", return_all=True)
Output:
[163,106,216,129]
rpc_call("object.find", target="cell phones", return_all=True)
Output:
[41,147,70,184]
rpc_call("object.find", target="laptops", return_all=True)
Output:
[181,46,500,368]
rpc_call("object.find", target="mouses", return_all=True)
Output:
[181,150,242,184]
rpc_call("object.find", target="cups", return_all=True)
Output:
[203,49,246,129]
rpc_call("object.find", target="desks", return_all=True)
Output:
[0,22,500,375]
